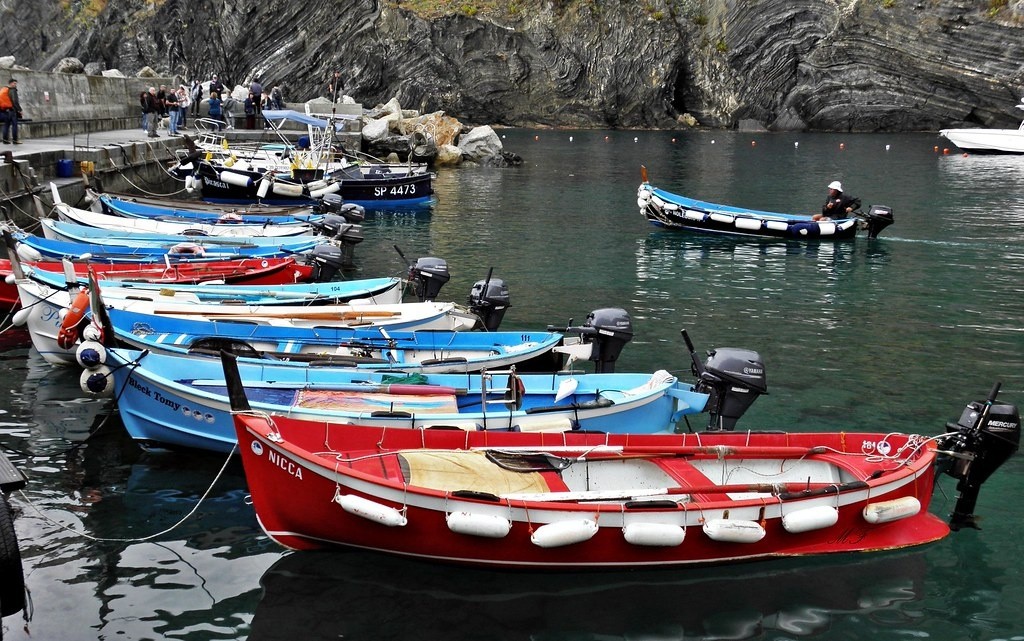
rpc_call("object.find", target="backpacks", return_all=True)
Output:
[0,85,16,111]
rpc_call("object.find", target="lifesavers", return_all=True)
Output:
[56,290,89,351]
[167,242,207,262]
[215,212,246,226]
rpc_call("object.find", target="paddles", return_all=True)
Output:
[484,446,825,471]
[172,376,471,399]
[458,480,866,504]
[122,283,322,298]
[149,305,402,321]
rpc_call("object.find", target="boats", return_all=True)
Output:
[0,107,770,457]
[213,345,1021,582]
[938,97,1024,155]
[633,159,899,244]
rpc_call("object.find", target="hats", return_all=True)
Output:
[828,181,844,192]
[160,84,167,88]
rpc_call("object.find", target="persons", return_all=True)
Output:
[330,70,344,104]
[813,181,861,222]
[0,78,23,144]
[139,73,282,137]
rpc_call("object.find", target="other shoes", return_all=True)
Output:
[184,126,188,130]
[159,114,162,120]
[148,133,160,137]
[226,125,231,130]
[264,127,272,131]
[13,139,23,144]
[189,112,194,117]
[231,127,234,130]
[196,113,201,117]
[3,140,11,144]
[175,132,181,134]
[163,113,169,116]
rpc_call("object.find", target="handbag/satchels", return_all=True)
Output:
[208,111,211,115]
